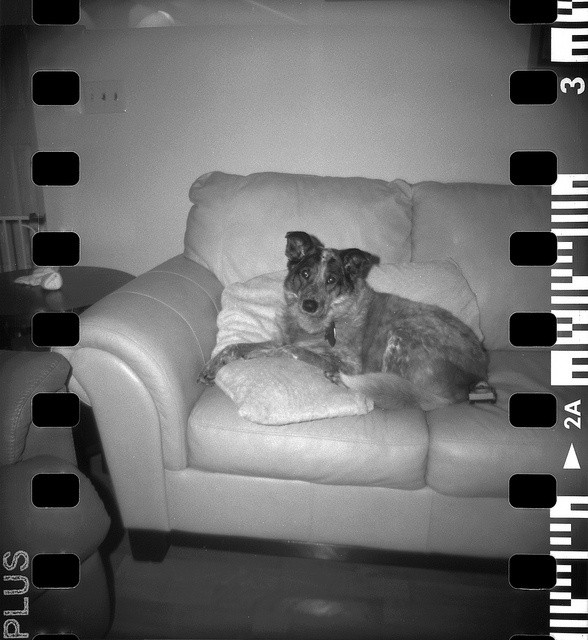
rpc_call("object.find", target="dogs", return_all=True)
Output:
[195,228,491,412]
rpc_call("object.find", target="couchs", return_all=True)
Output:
[50,172,587,563]
[0,348,114,639]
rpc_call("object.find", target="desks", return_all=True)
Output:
[0,267,137,324]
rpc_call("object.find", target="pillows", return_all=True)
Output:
[210,257,485,361]
[215,357,375,425]
